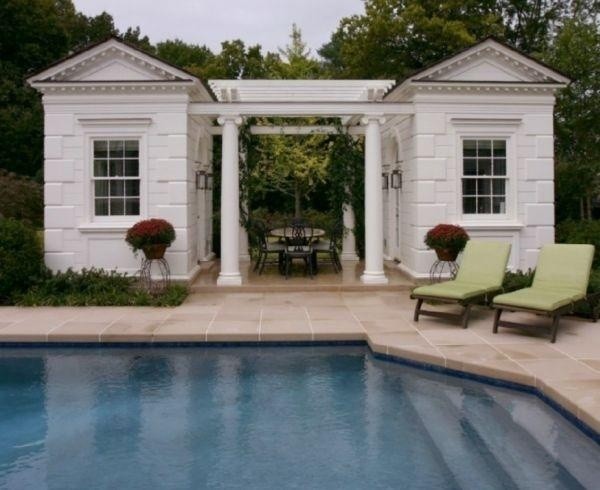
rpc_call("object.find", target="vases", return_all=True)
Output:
[141,245,166,260]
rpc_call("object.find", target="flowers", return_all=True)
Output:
[126,217,175,253]
[422,222,468,248]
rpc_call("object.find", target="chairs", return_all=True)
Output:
[250,215,346,280]
[410,240,596,343]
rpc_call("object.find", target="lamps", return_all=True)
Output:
[381,159,404,189]
[193,161,212,190]
